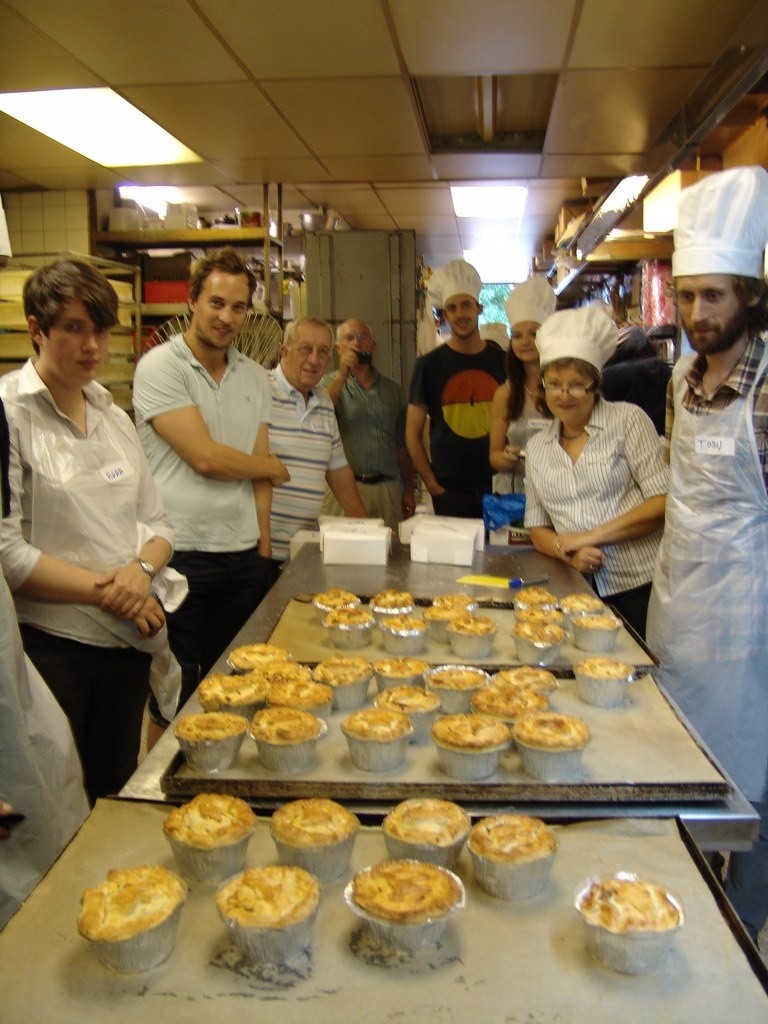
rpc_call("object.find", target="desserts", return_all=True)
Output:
[575,870,684,977]
[75,792,561,973]
[171,642,634,782]
[313,585,621,665]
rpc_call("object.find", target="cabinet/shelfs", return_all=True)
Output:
[89,226,286,359]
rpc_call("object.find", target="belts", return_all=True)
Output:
[358,471,391,485]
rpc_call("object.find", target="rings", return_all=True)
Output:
[590,564,593,571]
[556,544,560,549]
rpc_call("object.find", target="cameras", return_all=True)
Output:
[508,449,526,461]
[355,351,373,365]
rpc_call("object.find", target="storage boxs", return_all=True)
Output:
[318,516,385,550]
[419,516,486,552]
[399,514,428,545]
[409,523,478,567]
[322,526,393,566]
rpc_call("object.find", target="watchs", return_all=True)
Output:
[130,557,157,580]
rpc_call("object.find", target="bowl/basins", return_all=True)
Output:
[300,213,325,233]
[78,591,686,978]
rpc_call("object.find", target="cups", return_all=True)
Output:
[235,206,261,228]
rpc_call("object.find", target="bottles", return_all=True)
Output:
[197,217,206,230]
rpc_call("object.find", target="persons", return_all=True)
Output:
[132,246,290,728]
[645,164,768,954]
[0,260,177,814]
[266,316,370,587]
[489,278,559,496]
[315,319,415,550]
[0,206,90,942]
[404,258,510,518]
[525,303,668,643]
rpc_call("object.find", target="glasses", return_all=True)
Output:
[542,377,598,399]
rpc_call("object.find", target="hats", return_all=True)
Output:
[504,280,556,329]
[669,165,768,280]
[428,261,482,306]
[534,306,618,373]
[479,323,510,352]
